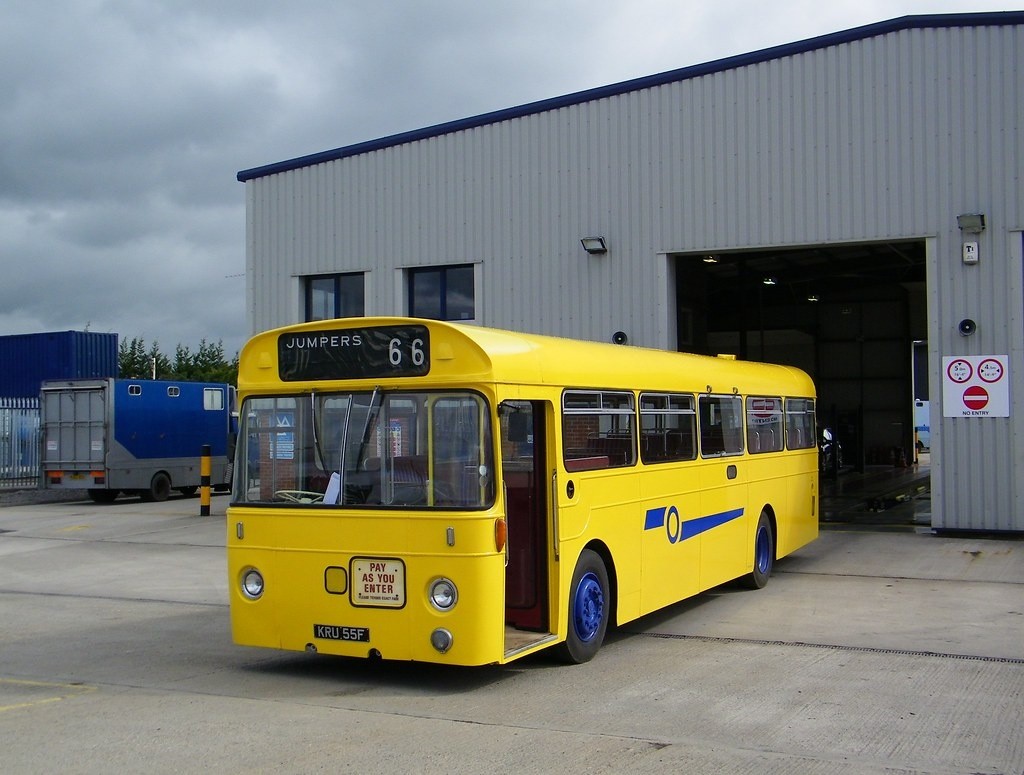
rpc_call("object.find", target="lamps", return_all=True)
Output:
[580,236,607,255]
[956,212,986,234]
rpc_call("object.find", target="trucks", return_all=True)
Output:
[38,378,261,504]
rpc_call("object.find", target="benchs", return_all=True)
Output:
[563,423,807,472]
[385,451,439,498]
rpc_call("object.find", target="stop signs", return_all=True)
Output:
[750,397,773,418]
[962,386,990,410]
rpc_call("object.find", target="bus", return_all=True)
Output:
[914,399,930,452]
[225,316,820,668]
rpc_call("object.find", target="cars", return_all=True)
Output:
[807,428,843,472]
